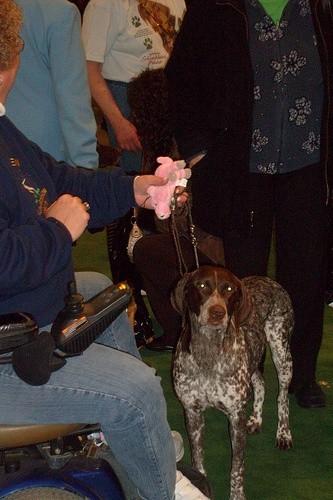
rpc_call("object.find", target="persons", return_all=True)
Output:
[0,1,188,500]
[10,0,333,409]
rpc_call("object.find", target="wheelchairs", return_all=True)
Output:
[0,279,214,500]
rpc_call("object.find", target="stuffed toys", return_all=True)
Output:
[148,156,191,219]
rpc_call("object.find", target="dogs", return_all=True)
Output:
[125,67,173,151]
[172,266,296,500]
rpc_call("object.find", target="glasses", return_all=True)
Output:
[15,35,24,54]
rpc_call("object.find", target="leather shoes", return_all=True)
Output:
[289,379,326,408]
[145,334,180,353]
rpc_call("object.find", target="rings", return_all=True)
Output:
[83,202,91,210]
